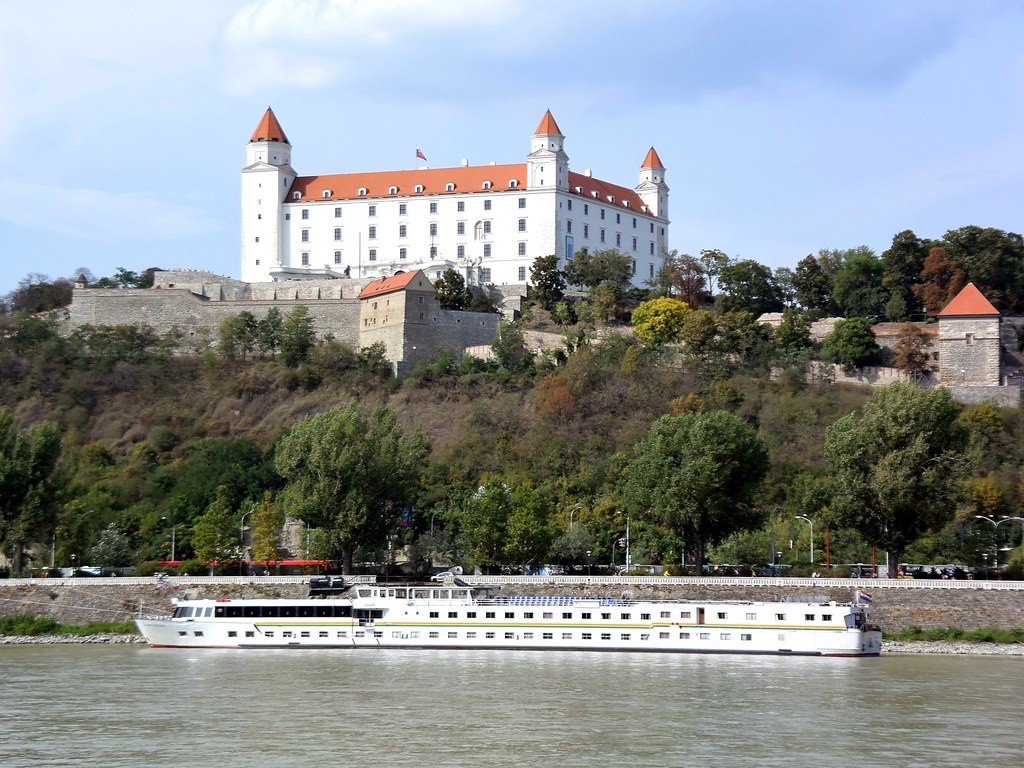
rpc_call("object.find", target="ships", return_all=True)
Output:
[133,585,882,656]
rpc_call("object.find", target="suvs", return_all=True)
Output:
[430,571,455,582]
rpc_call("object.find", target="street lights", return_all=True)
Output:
[796,516,813,563]
[162,517,174,561]
